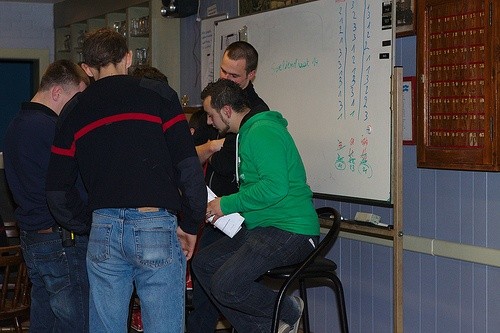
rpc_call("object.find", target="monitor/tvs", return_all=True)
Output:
[1,59,39,152]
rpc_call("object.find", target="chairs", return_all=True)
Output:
[229,206,350,332]
[0,244,33,333]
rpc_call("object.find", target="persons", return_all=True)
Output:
[187,39,275,332]
[186,78,320,332]
[3,57,92,333]
[46,26,209,332]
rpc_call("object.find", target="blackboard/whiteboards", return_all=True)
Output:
[198,11,229,93]
[211,0,396,207]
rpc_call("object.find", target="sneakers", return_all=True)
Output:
[277,295,303,333]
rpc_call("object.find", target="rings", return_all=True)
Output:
[210,211,213,216]
